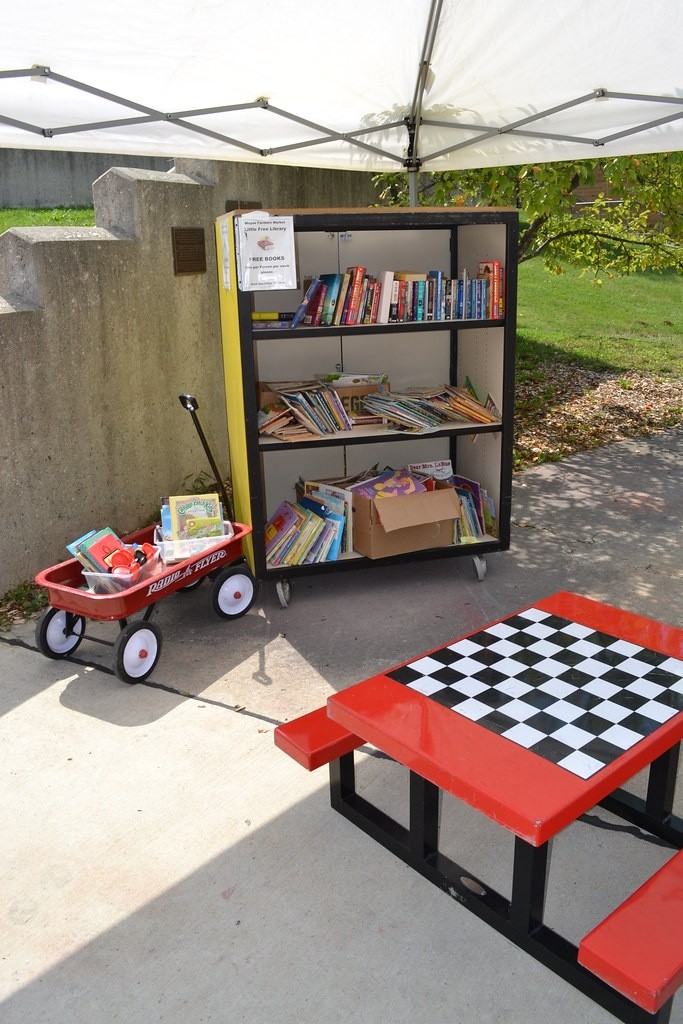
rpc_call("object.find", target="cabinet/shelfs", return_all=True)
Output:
[215,205,524,608]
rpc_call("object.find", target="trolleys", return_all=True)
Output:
[34,394,261,684]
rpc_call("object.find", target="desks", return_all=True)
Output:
[325,589,683,953]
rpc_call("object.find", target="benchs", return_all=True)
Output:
[274,704,409,866]
[529,815,683,1024]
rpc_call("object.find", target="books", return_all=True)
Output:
[67,527,127,593]
[257,373,502,441]
[265,459,497,566]
[157,494,232,563]
[251,261,506,329]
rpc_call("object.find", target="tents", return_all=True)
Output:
[0,1,683,207]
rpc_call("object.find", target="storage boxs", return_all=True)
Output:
[331,383,390,411]
[335,481,462,560]
[154,520,235,565]
[81,543,161,594]
